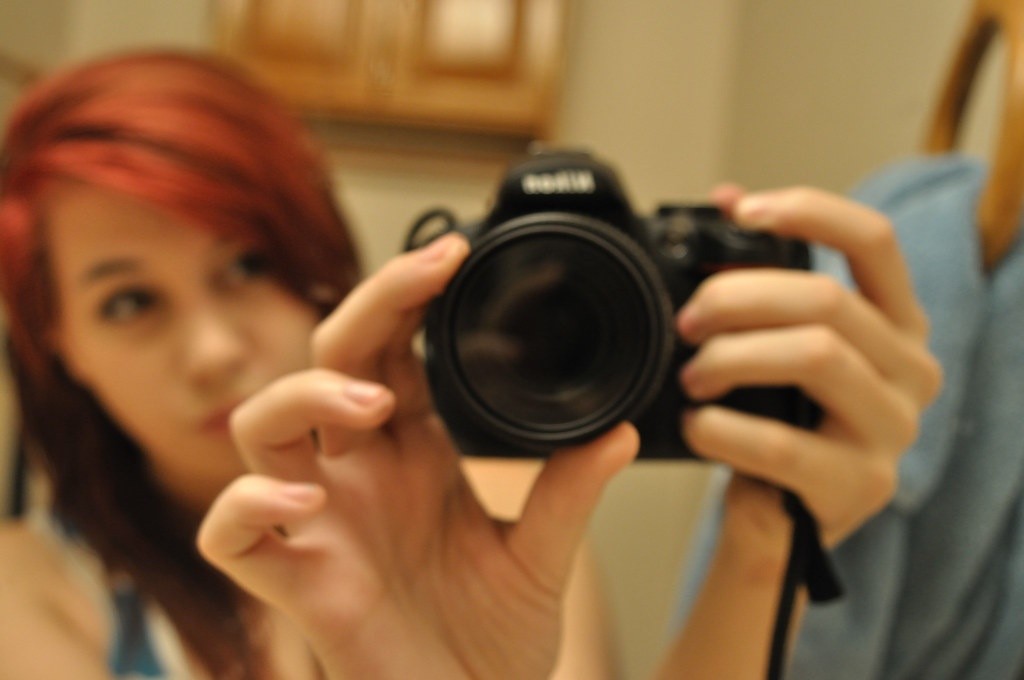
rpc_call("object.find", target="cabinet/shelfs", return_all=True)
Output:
[207,2,568,138]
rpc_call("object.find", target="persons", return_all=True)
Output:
[0,47,942,679]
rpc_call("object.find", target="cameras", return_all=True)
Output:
[424,146,827,461]
